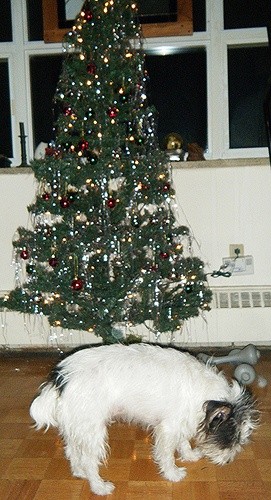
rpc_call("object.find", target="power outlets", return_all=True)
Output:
[229,244,244,258]
[222,256,255,277]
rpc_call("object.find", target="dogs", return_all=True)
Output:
[28,340,266,496]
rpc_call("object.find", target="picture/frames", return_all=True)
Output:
[41,0,193,44]
